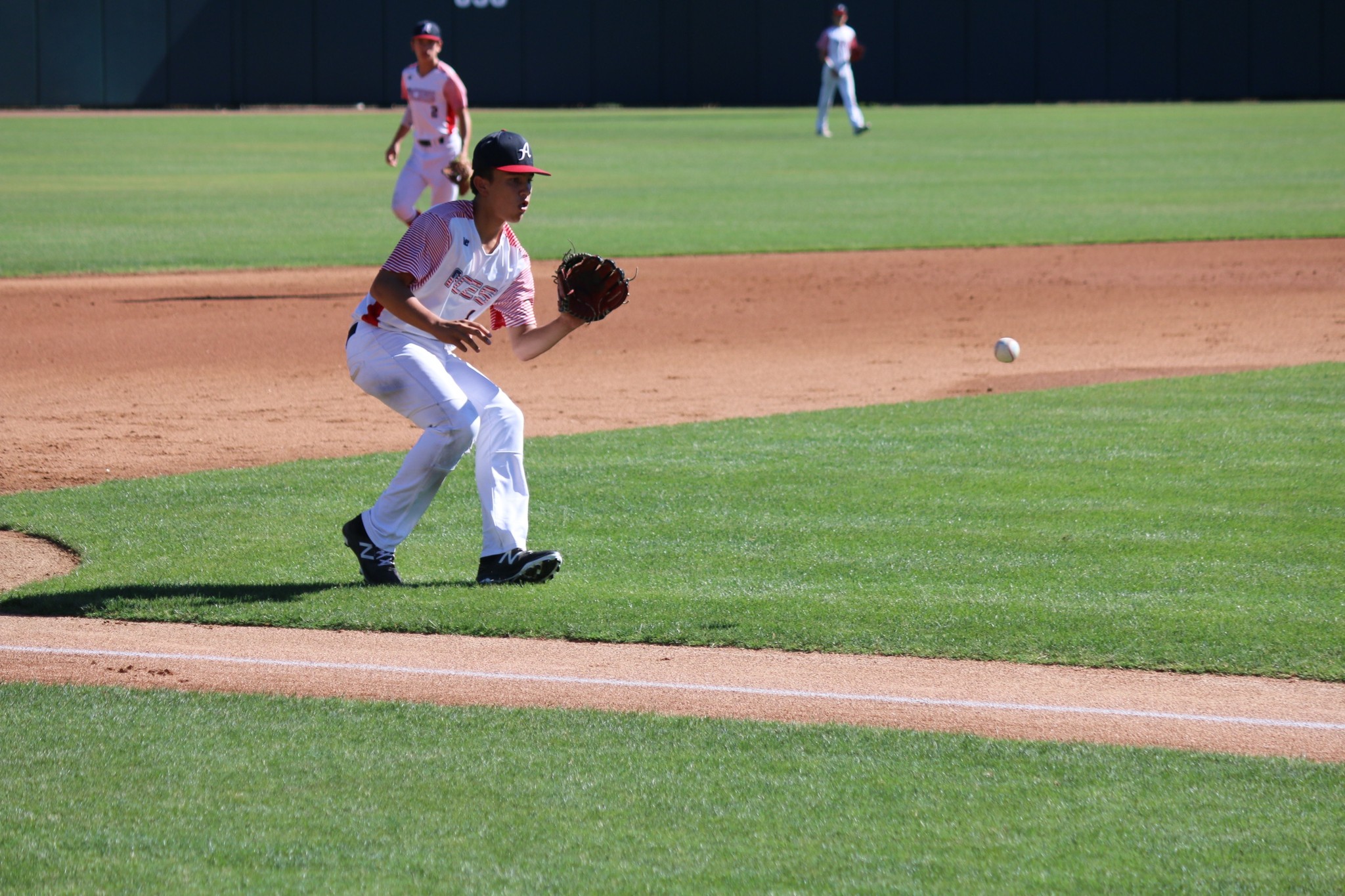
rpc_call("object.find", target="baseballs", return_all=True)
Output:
[995,338,1020,363]
[356,102,366,111]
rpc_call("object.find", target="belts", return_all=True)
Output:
[417,138,445,147]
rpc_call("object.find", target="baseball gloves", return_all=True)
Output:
[555,250,628,324]
[441,160,474,194]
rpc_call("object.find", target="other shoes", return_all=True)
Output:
[816,129,832,138]
[854,124,870,134]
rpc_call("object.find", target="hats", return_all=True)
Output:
[472,129,551,176]
[412,19,442,42]
[833,4,848,16]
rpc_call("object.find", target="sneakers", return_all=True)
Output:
[341,514,401,585]
[476,547,563,583]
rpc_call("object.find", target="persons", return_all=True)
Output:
[384,21,470,229]
[816,8,873,139]
[344,127,637,585]
